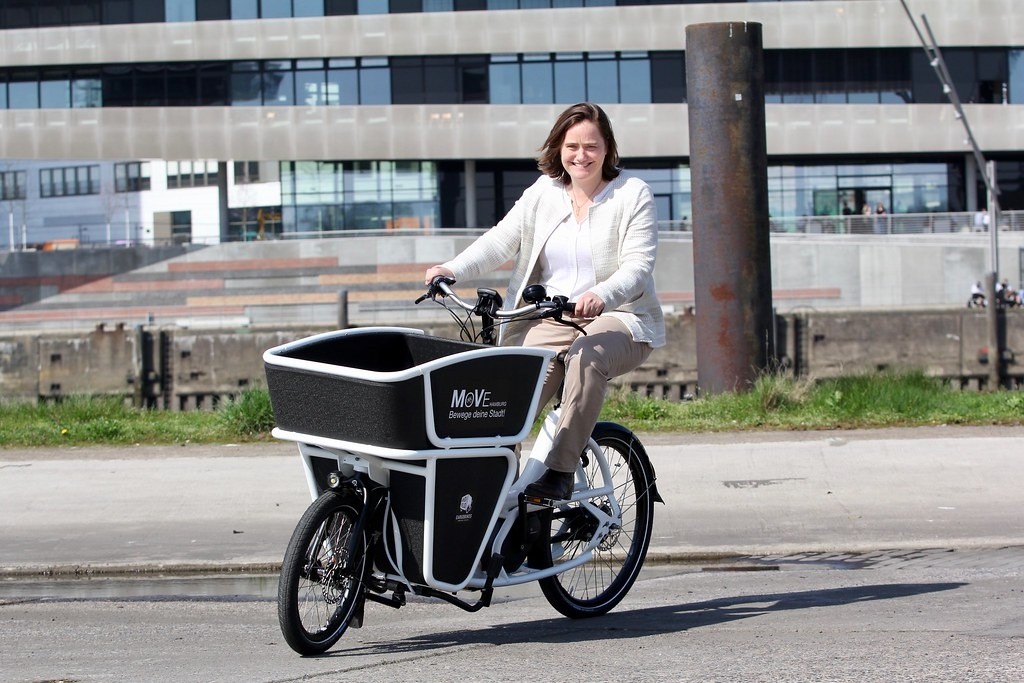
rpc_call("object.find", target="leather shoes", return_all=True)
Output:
[524,468,574,500]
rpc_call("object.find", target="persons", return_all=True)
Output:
[923,211,936,232]
[995,279,1024,308]
[425,102,665,502]
[842,204,852,232]
[862,204,872,232]
[972,281,985,308]
[874,203,888,233]
[975,210,986,231]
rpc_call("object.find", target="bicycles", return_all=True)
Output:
[262,274,665,656]
